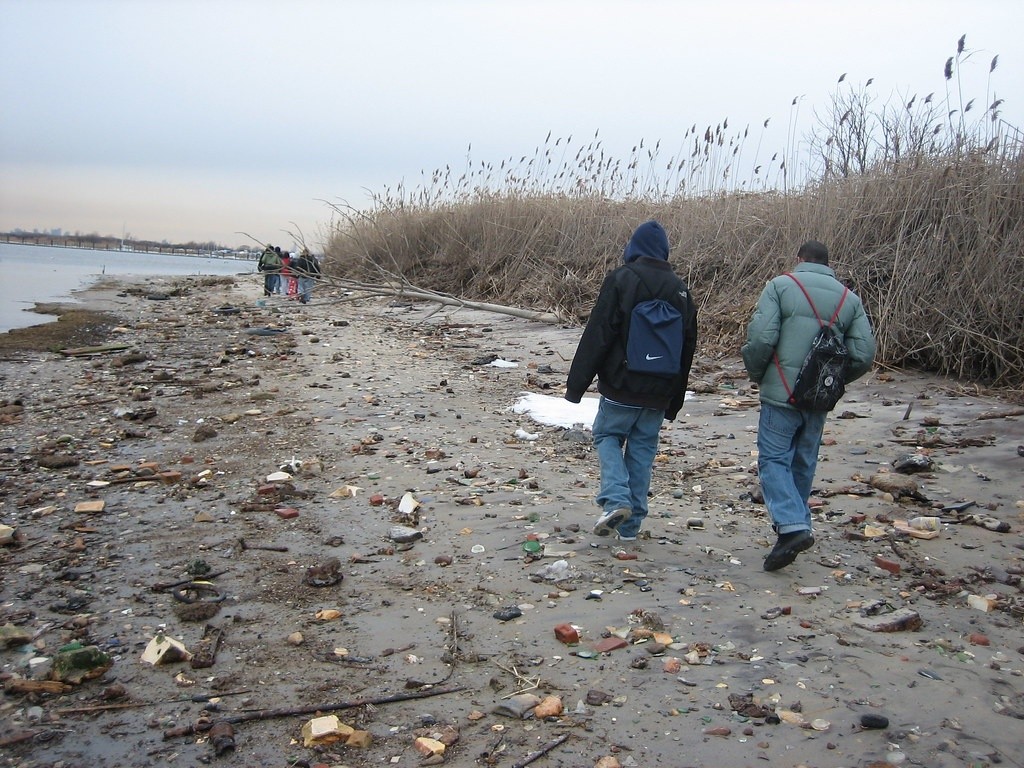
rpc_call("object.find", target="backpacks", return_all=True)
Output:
[772,271,849,412]
[620,262,684,378]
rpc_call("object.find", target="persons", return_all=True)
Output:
[741,242,878,572]
[258,244,321,304]
[564,222,697,542]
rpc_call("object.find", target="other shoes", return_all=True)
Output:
[763,530,814,571]
[299,298,307,304]
[615,529,635,542]
[592,508,631,536]
